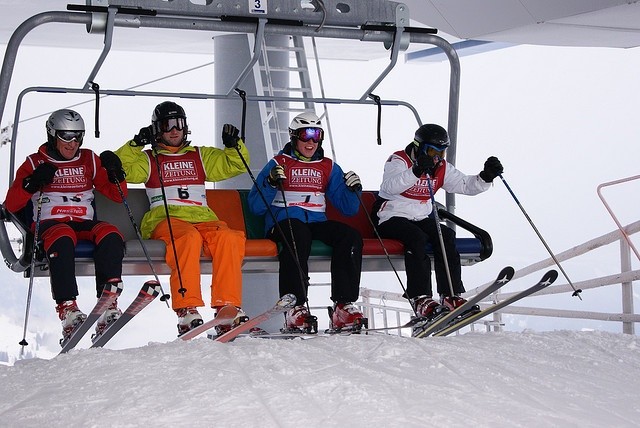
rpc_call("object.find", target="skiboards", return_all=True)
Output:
[238,327,367,338]
[56,278,160,354]
[416,266,558,338]
[179,294,298,343]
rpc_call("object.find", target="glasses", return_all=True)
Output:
[413,138,448,162]
[156,116,186,132]
[46,121,84,142]
[289,127,324,143]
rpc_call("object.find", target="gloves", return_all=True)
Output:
[412,153,434,178]
[100,150,126,184]
[480,156,504,182]
[343,171,362,192]
[268,164,287,188]
[21,163,57,193]
[222,124,240,147]
[133,125,153,145]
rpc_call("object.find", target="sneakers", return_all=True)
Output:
[331,300,363,330]
[284,305,313,329]
[56,298,87,336]
[177,306,204,334]
[410,294,450,321]
[438,293,479,326]
[95,299,122,332]
[214,309,249,336]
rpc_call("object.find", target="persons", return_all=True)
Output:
[3,107,129,336]
[248,111,364,332]
[370,124,505,321]
[112,101,249,336]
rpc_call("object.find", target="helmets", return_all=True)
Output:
[152,102,189,139]
[289,112,324,150]
[45,108,86,151]
[413,123,450,158]
